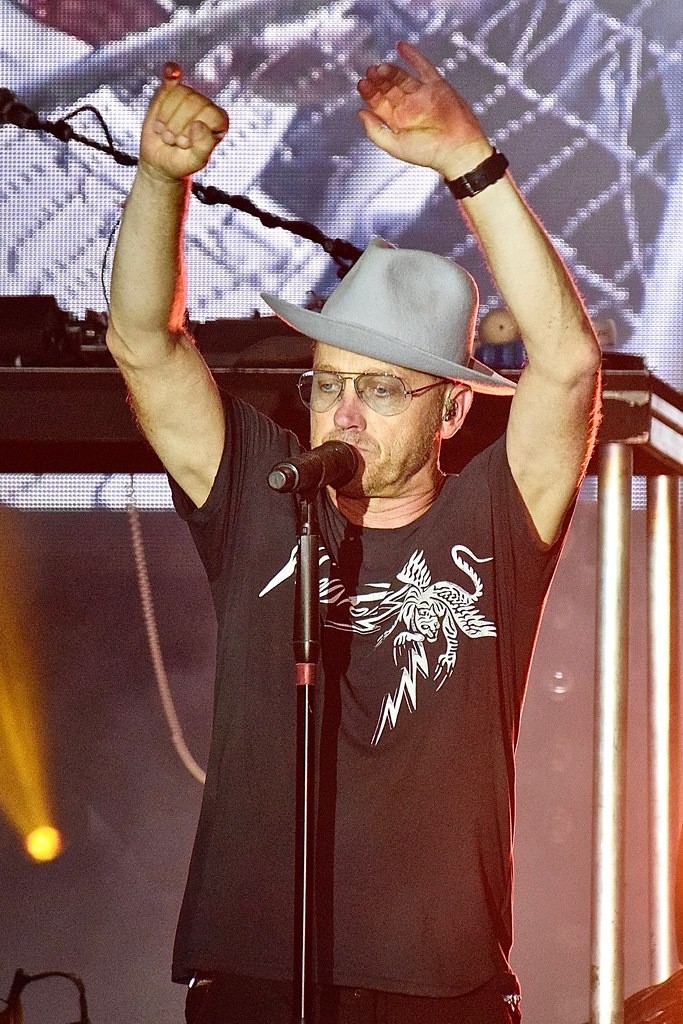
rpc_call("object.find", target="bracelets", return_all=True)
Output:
[444,146,509,201]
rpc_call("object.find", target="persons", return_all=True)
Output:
[106,45,607,1024]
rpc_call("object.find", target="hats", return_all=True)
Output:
[260,234,519,396]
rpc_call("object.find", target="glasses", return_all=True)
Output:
[296,369,451,417]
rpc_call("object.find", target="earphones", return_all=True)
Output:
[443,399,457,421]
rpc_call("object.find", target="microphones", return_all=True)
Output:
[0,87,39,130]
[267,440,358,494]
